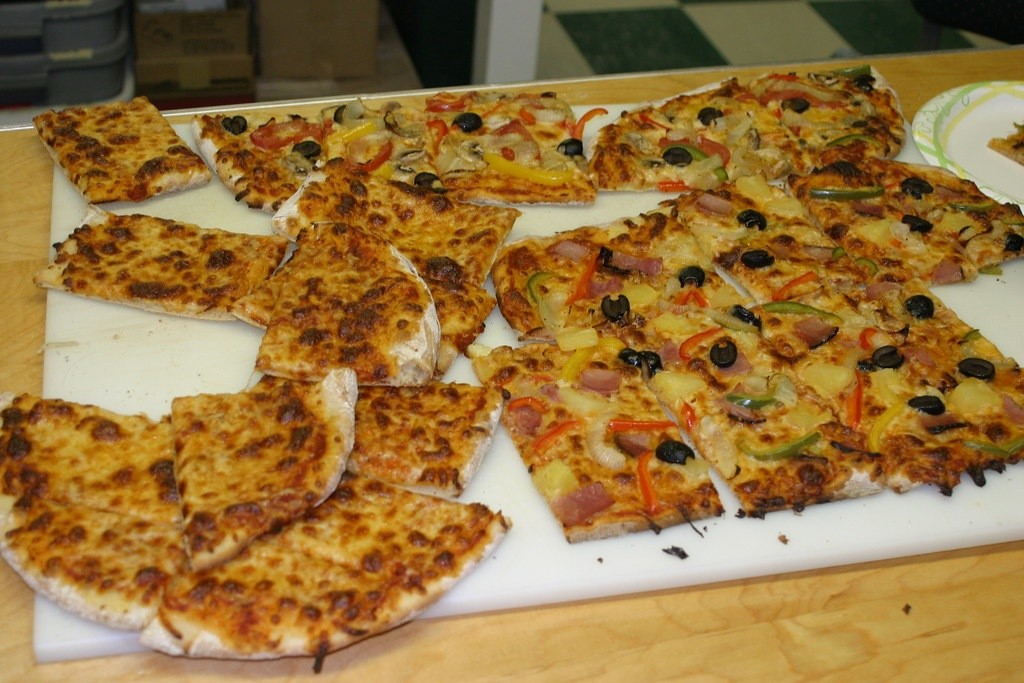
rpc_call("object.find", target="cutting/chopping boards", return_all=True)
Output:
[28,102,1024,664]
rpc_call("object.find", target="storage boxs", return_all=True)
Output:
[132,7,248,56]
[137,54,248,86]
[0,10,128,103]
[0,0,122,52]
[253,0,380,79]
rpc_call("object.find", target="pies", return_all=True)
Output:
[0,90,522,661]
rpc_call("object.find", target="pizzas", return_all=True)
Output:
[190,59,1024,544]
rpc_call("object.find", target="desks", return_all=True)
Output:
[0,41,1024,683]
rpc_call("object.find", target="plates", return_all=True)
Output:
[911,79,1024,216]
